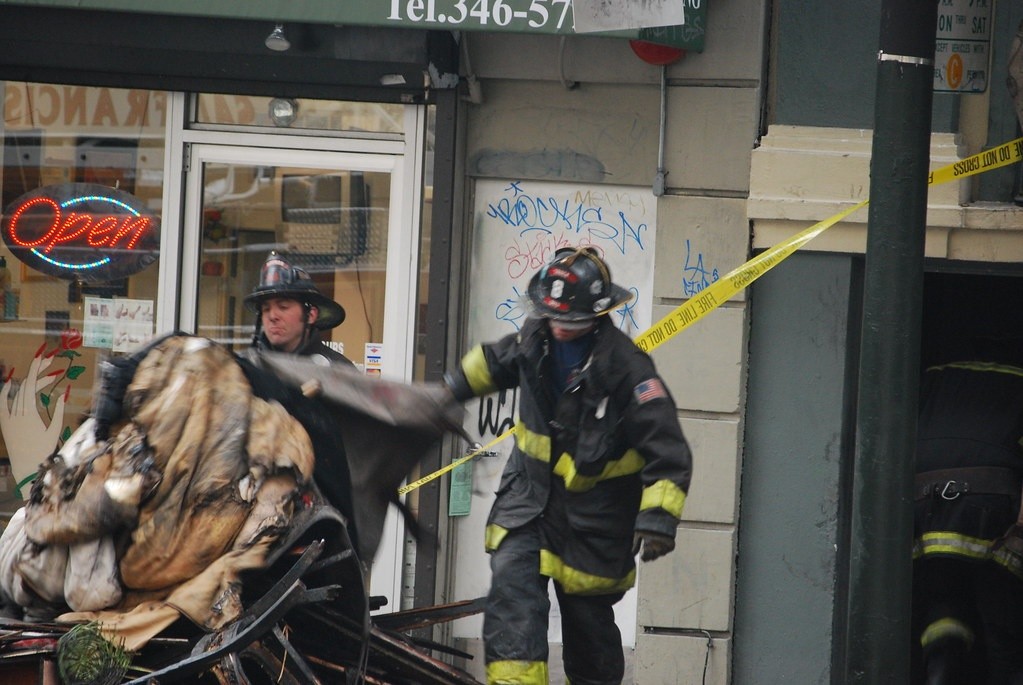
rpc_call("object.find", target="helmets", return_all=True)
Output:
[242,249,346,330]
[528,245,634,322]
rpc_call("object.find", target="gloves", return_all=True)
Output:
[631,530,675,561]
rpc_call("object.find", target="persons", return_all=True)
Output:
[245,250,358,370]
[915,329,1022,684]
[426,251,692,685]
